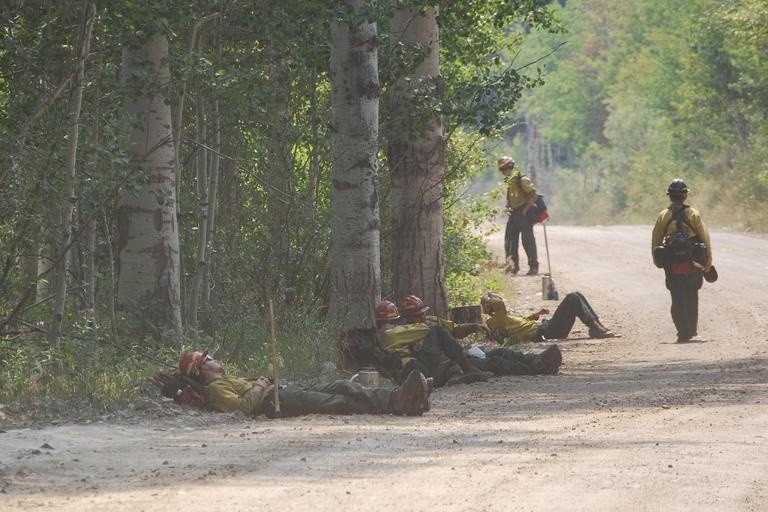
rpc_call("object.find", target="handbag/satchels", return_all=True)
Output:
[528,195,546,223]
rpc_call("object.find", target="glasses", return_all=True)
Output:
[203,355,212,364]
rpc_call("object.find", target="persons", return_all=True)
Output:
[178,349,438,420]
[497,155,541,275]
[651,178,713,344]
[374,299,495,386]
[399,293,563,377]
[480,290,622,344]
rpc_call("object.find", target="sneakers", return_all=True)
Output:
[532,345,561,374]
[677,330,696,342]
[392,370,428,416]
[447,368,493,384]
[589,328,614,338]
[526,266,537,274]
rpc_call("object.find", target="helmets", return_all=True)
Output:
[400,295,428,316]
[482,295,495,312]
[499,157,514,170]
[180,349,208,376]
[376,301,400,320]
[667,180,687,197]
